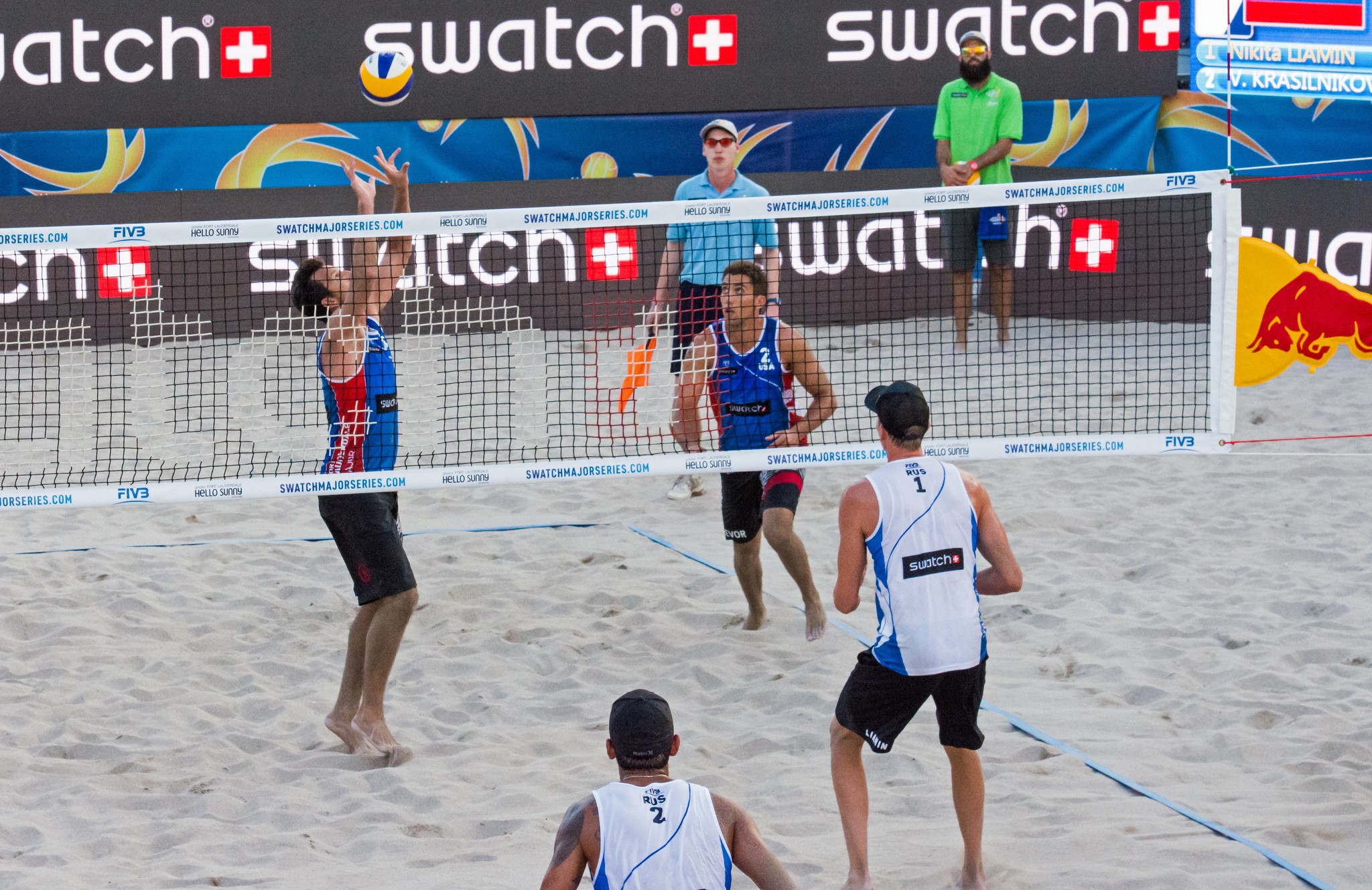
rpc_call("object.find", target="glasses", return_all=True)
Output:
[703,137,736,148]
[960,44,987,57]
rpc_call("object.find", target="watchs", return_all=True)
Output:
[968,160,979,172]
[767,298,782,305]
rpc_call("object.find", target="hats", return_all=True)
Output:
[700,119,739,139]
[958,30,989,52]
[609,688,674,755]
[864,380,930,440]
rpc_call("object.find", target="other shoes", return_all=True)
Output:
[667,473,705,499]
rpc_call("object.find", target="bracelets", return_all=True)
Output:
[651,301,664,306]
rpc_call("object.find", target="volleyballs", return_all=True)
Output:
[358,50,414,107]
[941,160,981,188]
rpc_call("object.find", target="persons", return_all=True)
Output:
[645,119,782,501]
[832,382,1024,890]
[932,30,1024,349]
[286,145,420,757]
[539,688,795,890]
[672,258,840,642]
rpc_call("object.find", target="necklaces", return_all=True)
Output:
[624,775,667,780]
